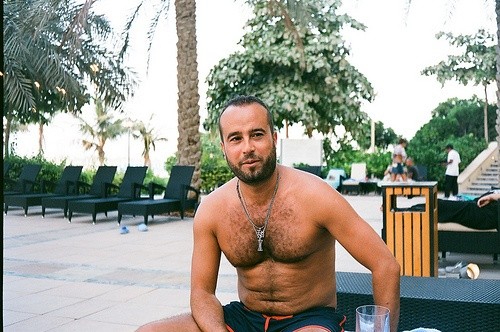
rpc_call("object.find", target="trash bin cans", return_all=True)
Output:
[381,181,440,277]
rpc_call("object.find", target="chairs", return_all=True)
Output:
[67,166,148,225]
[3,164,42,210]
[117,165,200,225]
[3,166,83,217]
[381,198,500,264]
[295,162,428,196]
[41,166,117,219]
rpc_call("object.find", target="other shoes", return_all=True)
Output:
[136,223,148,232]
[120,226,130,234]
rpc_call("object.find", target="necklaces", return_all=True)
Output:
[236,167,279,251]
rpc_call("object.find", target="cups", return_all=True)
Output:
[355,305,390,332]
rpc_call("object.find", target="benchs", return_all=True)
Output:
[333,271,500,332]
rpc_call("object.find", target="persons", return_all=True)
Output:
[445,144,461,198]
[415,190,500,230]
[391,138,407,182]
[405,159,417,181]
[136,97,400,332]
